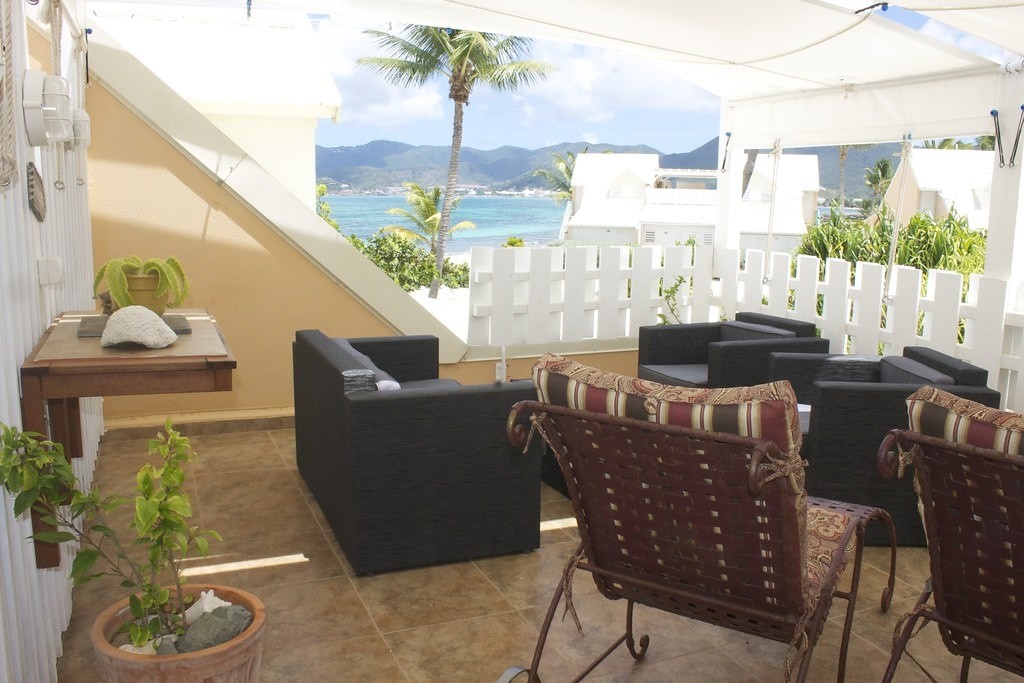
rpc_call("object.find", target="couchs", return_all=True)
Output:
[638,310,829,388]
[292,328,544,576]
[767,342,1002,549]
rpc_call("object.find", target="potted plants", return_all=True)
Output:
[92,255,189,319]
[1,415,266,683]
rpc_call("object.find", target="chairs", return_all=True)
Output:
[500,352,900,683]
[878,383,1024,683]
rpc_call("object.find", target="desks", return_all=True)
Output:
[17,307,238,573]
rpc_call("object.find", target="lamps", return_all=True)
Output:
[22,67,72,144]
[64,107,90,148]
